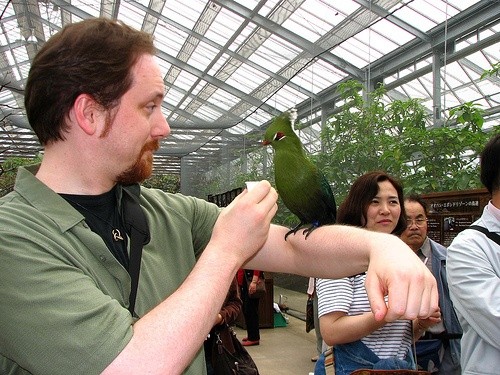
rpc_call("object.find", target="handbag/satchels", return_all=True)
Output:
[349,368,433,375]
[413,337,445,373]
[247,278,266,300]
[204,319,261,375]
[305,296,314,333]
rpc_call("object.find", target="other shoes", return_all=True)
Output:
[242,336,248,341]
[241,339,259,346]
[311,355,320,362]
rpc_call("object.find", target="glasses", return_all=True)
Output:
[406,218,429,227]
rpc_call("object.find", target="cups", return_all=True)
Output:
[244,182,260,194]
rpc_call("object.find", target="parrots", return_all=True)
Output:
[263,115,338,240]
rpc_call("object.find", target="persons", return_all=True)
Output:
[215,172,463,375]
[446,134,500,375]
[0,18,439,375]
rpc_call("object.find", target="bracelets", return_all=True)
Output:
[251,281,257,284]
